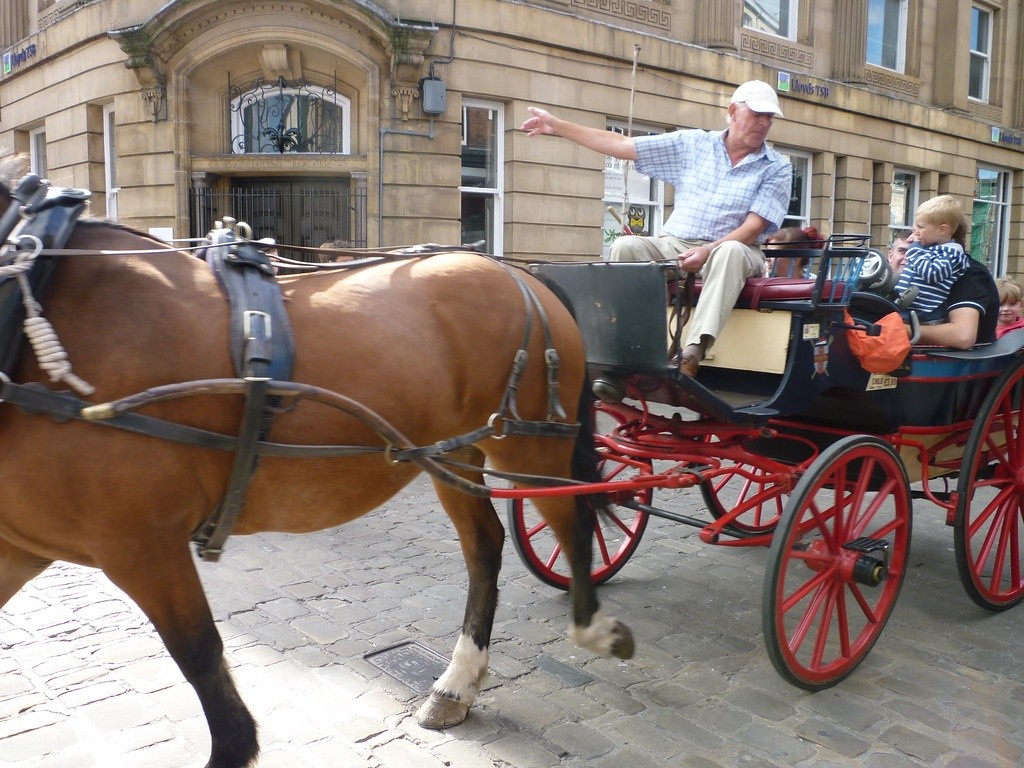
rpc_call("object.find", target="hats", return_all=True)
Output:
[732,80,784,120]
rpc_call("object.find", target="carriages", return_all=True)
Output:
[1,172,1024,768]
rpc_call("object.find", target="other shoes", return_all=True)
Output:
[667,352,698,378]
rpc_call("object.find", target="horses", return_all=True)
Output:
[0,180,636,768]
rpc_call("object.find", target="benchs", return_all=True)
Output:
[667,234,870,377]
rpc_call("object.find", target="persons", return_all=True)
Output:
[802,193,1024,436]
[520,79,793,380]
